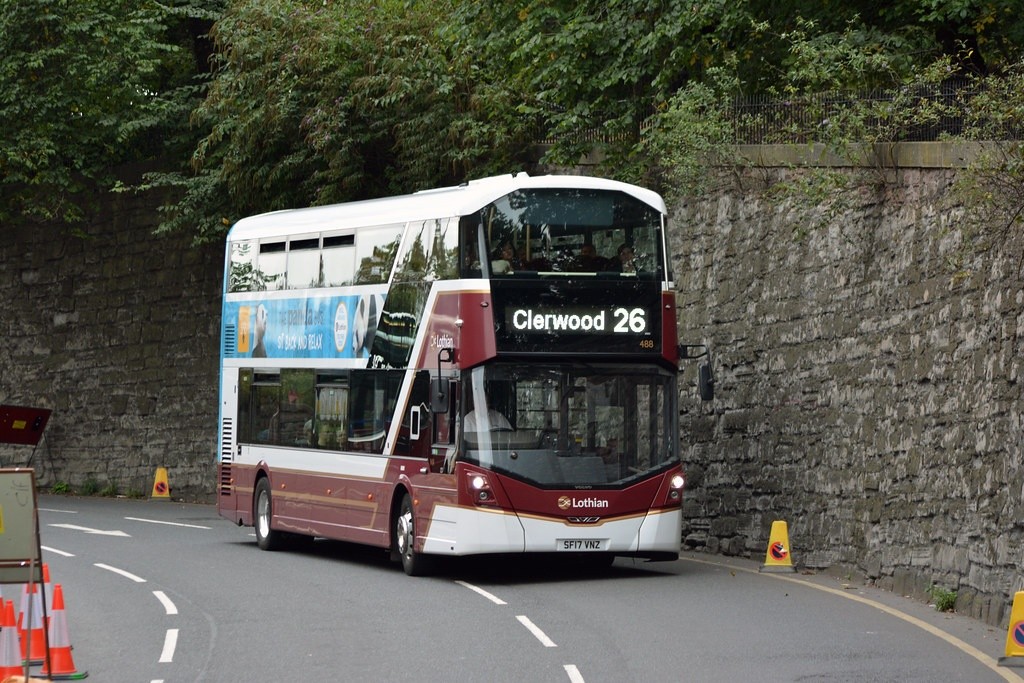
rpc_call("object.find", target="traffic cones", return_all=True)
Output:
[0,563,89,683]
[759,520,796,574]
[996,591,1024,668]
[149,466,172,502]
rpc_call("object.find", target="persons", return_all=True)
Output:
[465,240,646,273]
[296,419,373,453]
[464,393,513,432]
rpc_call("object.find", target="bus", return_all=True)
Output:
[212,174,720,577]
